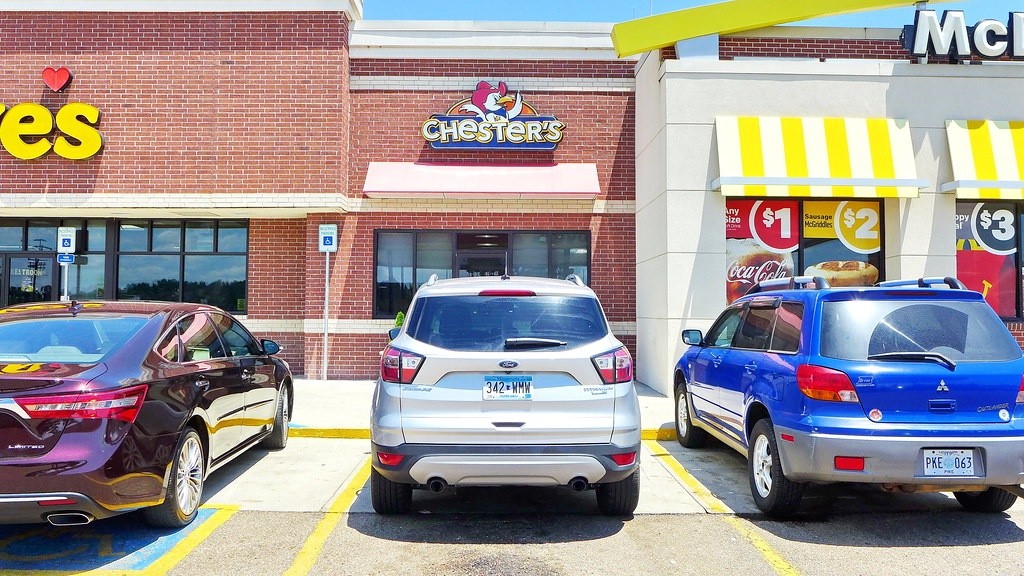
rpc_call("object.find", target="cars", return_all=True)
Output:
[0,300,294,529]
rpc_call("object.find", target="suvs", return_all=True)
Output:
[371,273,641,515]
[673,276,1024,522]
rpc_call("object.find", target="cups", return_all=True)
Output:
[726,238,795,307]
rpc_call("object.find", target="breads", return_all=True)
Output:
[803,260,878,287]
